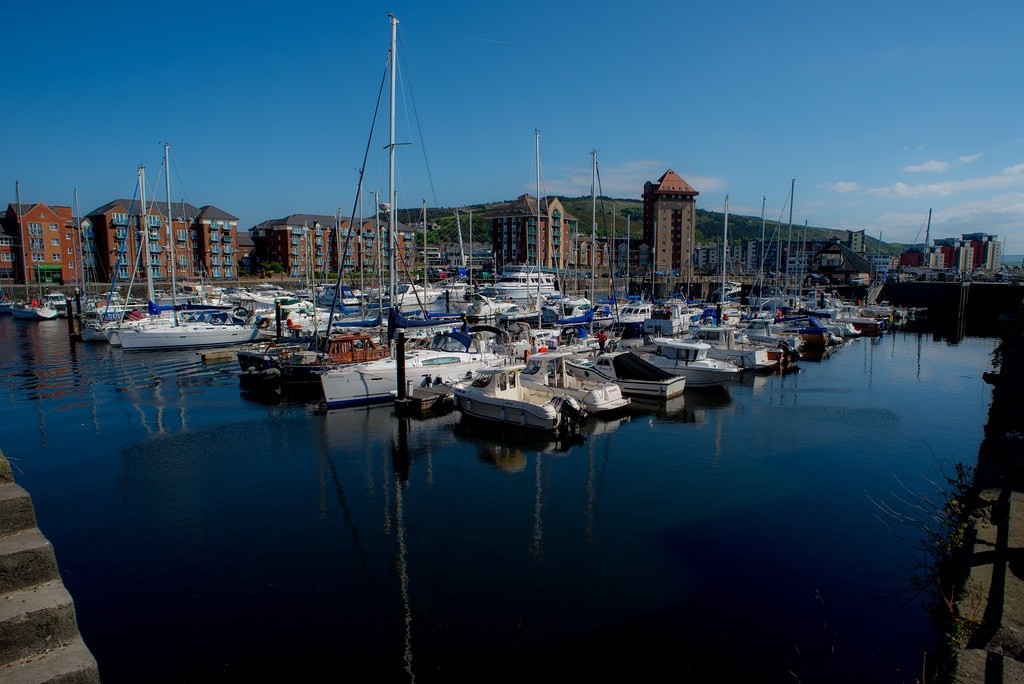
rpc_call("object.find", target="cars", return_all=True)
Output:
[849,277,865,286]
[804,273,830,286]
[886,273,898,283]
[929,264,1024,285]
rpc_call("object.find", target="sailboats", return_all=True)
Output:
[0,254,23,314]
[9,180,59,319]
[68,11,659,370]
[659,179,929,361]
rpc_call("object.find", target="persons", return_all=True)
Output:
[595,330,608,354]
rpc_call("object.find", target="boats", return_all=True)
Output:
[578,352,688,401]
[279,349,518,414]
[43,293,67,318]
[639,335,740,386]
[519,351,632,416]
[452,358,566,432]
[682,326,777,372]
